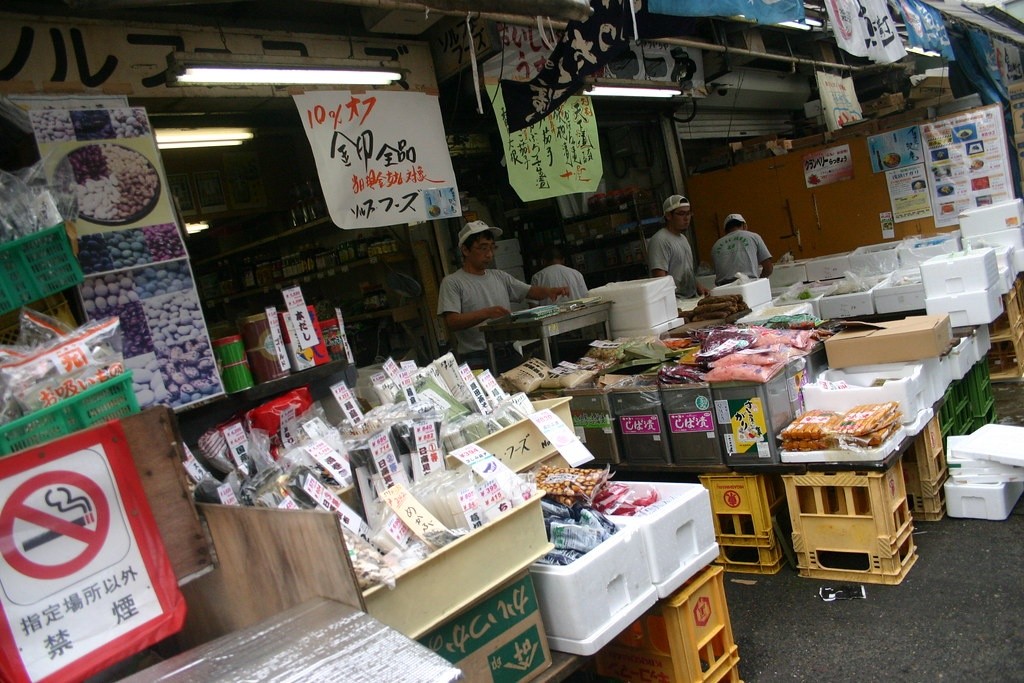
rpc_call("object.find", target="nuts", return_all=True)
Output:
[30,109,76,143]
[74,223,221,408]
[108,108,151,139]
[74,145,159,221]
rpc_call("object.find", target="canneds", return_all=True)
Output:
[368,240,400,257]
[212,312,292,394]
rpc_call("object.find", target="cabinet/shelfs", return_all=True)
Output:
[195,217,441,360]
[169,360,356,470]
[506,190,666,297]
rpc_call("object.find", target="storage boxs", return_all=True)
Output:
[0,197,1024,683]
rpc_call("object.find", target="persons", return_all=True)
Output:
[526,245,590,309]
[647,195,712,316]
[710,213,775,286]
[436,220,574,376]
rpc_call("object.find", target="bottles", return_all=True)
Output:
[284,176,326,228]
[214,227,402,314]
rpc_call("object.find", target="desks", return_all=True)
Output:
[479,303,611,378]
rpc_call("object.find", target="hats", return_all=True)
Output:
[458,220,504,248]
[660,194,691,223]
[724,214,746,231]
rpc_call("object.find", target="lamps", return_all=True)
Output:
[153,126,263,150]
[577,79,683,99]
[741,14,827,34]
[168,53,411,86]
